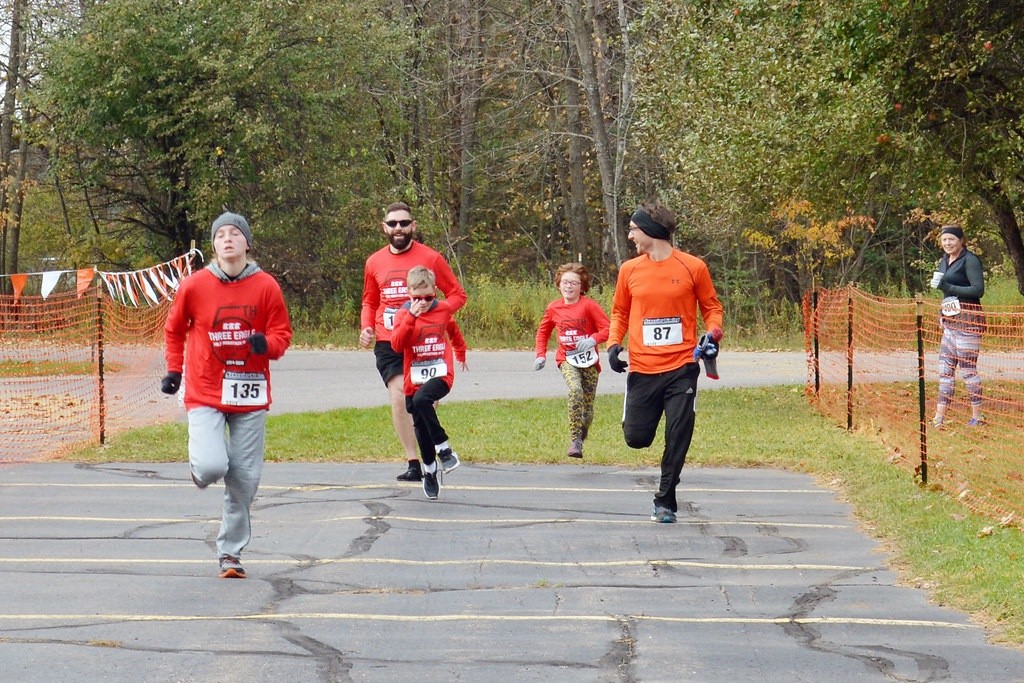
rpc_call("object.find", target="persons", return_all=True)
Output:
[534,263,610,458]
[607,205,724,523]
[160,211,293,578]
[391,264,469,500]
[931,224,987,429]
[360,202,467,482]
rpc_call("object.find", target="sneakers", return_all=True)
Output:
[965,414,987,428]
[567,438,583,458]
[424,459,439,500]
[397,467,424,482]
[437,448,460,474]
[929,420,943,430]
[651,504,677,523]
[219,556,246,578]
[190,472,208,488]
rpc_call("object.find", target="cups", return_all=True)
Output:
[933,271,944,281]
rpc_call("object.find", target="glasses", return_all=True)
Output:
[409,291,436,302]
[384,219,412,228]
[561,279,581,288]
[629,226,637,232]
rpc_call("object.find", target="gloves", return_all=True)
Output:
[534,358,545,371]
[161,372,182,394]
[608,344,628,374]
[247,333,267,354]
[930,276,943,289]
[699,335,720,361]
[575,338,596,352]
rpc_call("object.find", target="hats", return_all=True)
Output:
[211,212,252,252]
